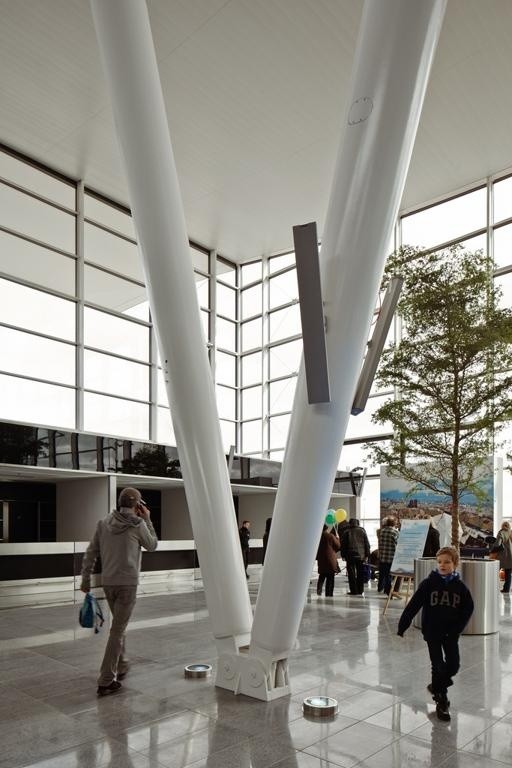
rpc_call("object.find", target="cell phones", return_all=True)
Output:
[136,500,143,510]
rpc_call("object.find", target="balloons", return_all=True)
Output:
[325,507,348,533]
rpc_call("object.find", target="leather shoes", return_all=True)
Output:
[499,589,509,594]
[347,591,363,595]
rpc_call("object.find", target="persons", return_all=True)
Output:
[240,521,251,579]
[491,522,512,593]
[376,518,399,598]
[378,516,399,593]
[316,524,342,599]
[396,547,474,722]
[344,519,371,596]
[81,488,158,696]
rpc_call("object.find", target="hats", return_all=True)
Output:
[118,487,146,504]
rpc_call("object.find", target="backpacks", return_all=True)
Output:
[77,592,103,634]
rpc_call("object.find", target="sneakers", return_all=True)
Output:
[96,680,122,696]
[426,684,451,721]
[116,663,130,681]
